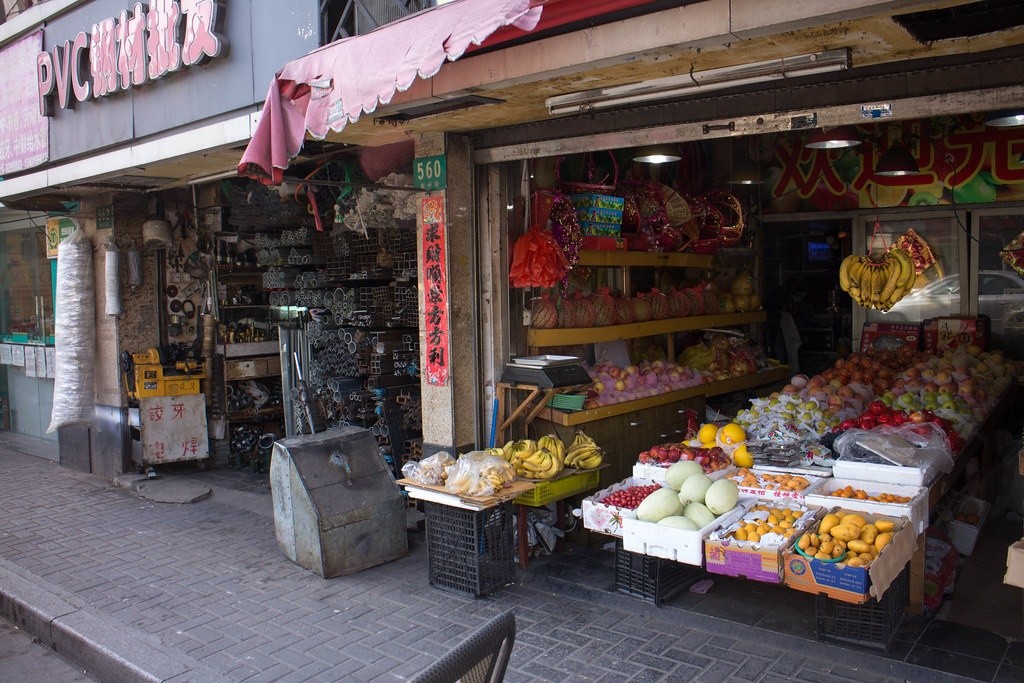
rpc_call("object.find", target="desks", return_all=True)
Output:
[496,382,593,449]
[220,357,283,439]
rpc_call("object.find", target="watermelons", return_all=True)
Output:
[530,286,719,329]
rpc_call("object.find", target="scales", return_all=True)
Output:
[501,352,593,389]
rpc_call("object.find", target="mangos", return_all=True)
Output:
[719,466,811,492]
[724,504,803,543]
[797,511,896,567]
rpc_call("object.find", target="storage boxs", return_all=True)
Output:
[923,319,990,358]
[575,208,622,225]
[577,236,627,252]
[577,222,620,237]
[704,499,823,584]
[423,501,516,595]
[633,461,736,483]
[581,476,665,536]
[512,469,599,507]
[929,461,992,555]
[805,478,929,540]
[1003,537,1024,589]
[783,506,919,605]
[815,562,909,653]
[613,537,713,608]
[860,322,922,356]
[832,432,936,486]
[569,194,624,211]
[720,468,824,505]
[623,496,760,566]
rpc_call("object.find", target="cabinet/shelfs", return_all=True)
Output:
[527,249,789,426]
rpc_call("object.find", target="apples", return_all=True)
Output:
[638,442,731,474]
[585,359,714,409]
[733,345,1017,452]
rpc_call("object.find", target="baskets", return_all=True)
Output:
[555,149,744,254]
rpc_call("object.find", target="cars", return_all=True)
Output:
[881,269,1024,332]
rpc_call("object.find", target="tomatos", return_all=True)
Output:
[600,484,662,509]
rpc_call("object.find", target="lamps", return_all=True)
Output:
[874,122,920,176]
[985,108,1024,127]
[725,136,768,184]
[544,46,852,116]
[632,142,683,164]
[805,125,864,149]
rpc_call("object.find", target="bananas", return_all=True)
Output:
[415,429,605,495]
[839,249,915,312]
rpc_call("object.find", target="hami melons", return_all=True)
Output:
[636,461,738,529]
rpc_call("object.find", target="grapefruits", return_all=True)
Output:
[718,268,759,313]
[679,424,755,468]
